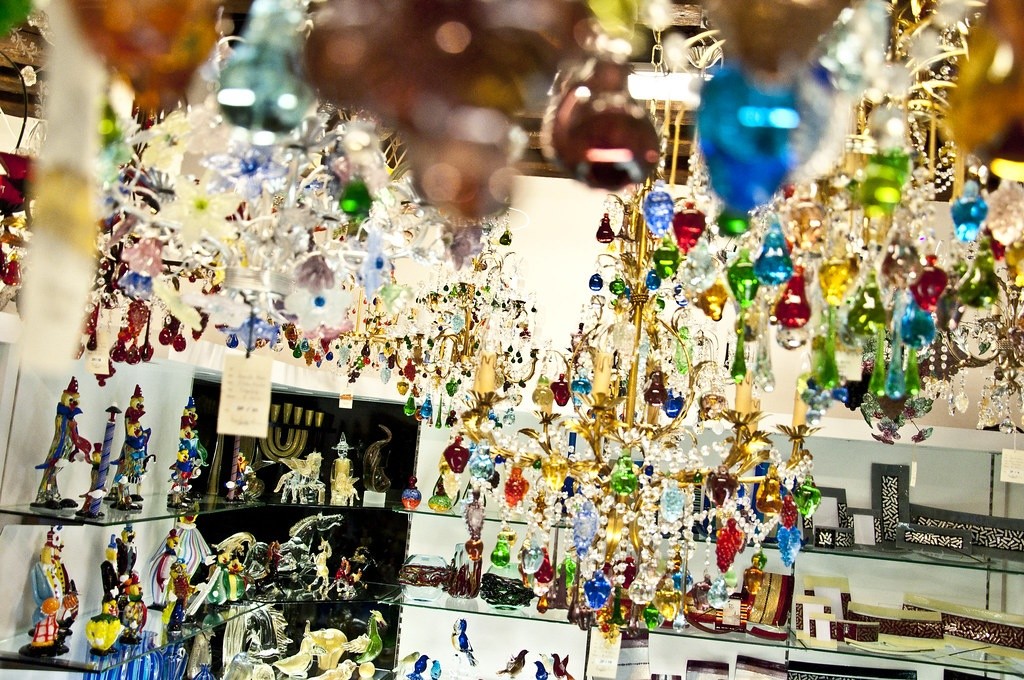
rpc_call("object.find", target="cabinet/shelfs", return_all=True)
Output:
[0,321,1024,680]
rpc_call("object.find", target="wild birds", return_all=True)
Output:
[222,653,263,680]
[392,652,441,680]
[273,637,326,677]
[340,609,387,664]
[451,618,478,668]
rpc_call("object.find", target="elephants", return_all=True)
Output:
[304,620,348,670]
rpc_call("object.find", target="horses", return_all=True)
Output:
[278,512,345,593]
